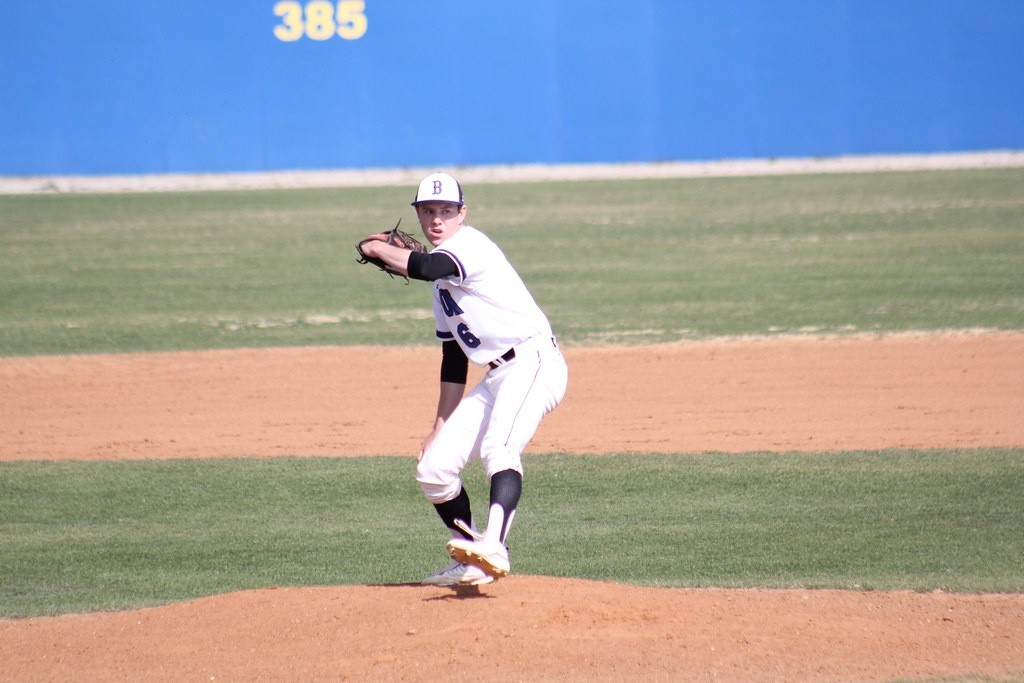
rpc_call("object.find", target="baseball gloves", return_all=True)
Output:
[355,229,427,277]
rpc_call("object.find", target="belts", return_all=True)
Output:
[488,347,516,369]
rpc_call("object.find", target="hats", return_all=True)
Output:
[411,171,463,206]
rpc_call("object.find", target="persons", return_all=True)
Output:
[353,172,568,586]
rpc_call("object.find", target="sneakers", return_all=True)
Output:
[421,560,495,587]
[445,519,510,578]
